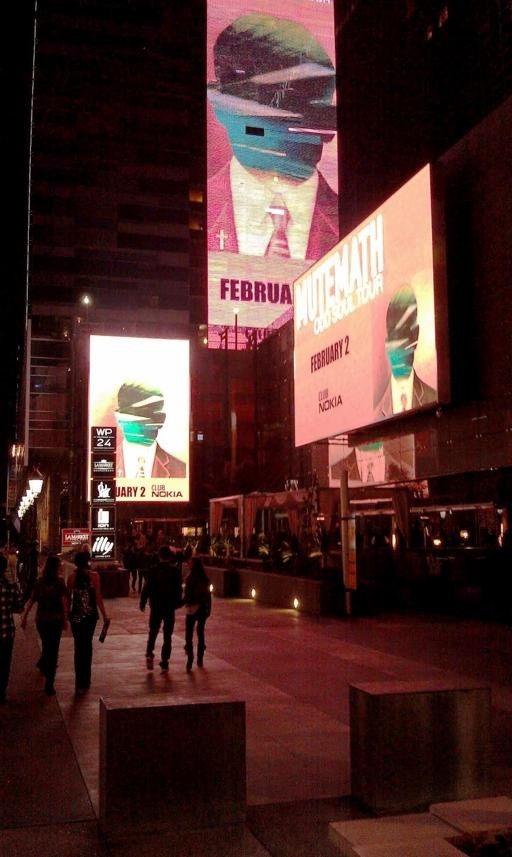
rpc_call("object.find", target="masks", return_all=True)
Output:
[388,345,415,376]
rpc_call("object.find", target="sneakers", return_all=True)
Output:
[160,662,168,669]
[187,655,203,669]
[146,654,154,670]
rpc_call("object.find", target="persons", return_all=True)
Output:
[183,557,212,670]
[0,551,110,705]
[121,543,192,592]
[139,546,183,670]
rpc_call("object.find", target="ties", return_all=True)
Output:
[367,463,375,482]
[401,394,407,410]
[135,457,145,478]
